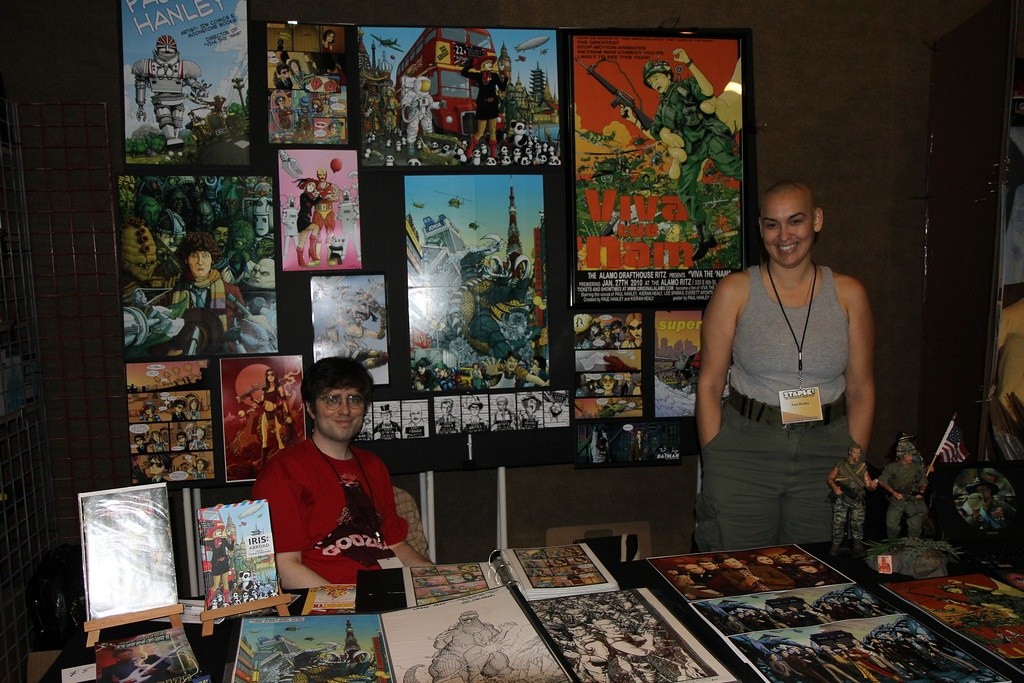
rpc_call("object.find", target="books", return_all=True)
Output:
[403,542,619,607]
[78,482,178,622]
[195,498,280,613]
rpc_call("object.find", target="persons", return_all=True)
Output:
[100,648,170,683]
[668,553,845,598]
[767,629,982,683]
[879,441,928,540]
[955,468,1016,530]
[696,180,873,549]
[252,357,434,589]
[827,445,878,554]
[899,431,934,478]
[724,597,883,634]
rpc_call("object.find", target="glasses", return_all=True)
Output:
[314,394,366,410]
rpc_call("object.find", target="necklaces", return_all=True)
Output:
[803,269,814,306]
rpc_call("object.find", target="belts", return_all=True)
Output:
[726,387,846,427]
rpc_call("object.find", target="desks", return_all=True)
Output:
[33,529,1024,683]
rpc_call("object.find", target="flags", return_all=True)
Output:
[940,424,969,462]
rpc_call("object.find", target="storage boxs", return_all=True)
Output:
[27,650,63,683]
